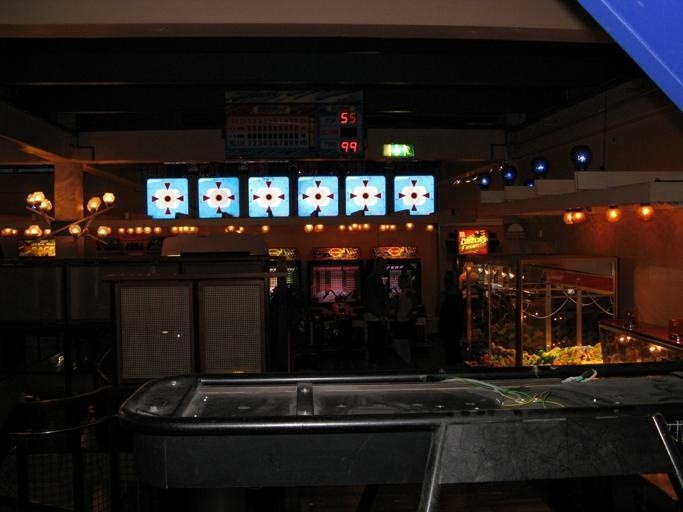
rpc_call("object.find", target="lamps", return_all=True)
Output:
[563,201,655,224]
[2,191,117,247]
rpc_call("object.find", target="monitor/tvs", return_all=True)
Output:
[457,227,488,256]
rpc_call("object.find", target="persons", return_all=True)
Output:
[394,274,418,330]
[436,268,468,376]
[458,262,480,289]
